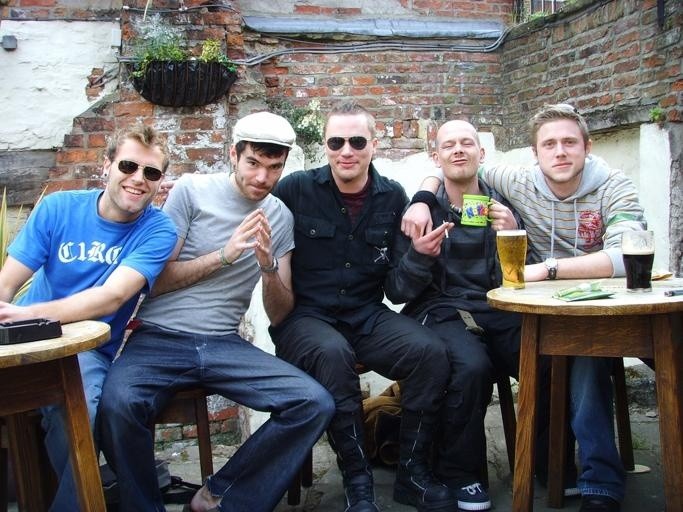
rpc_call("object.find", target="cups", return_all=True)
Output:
[621,229,656,292]
[495,229,528,290]
[460,194,495,227]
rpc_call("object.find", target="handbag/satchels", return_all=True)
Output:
[99,460,172,506]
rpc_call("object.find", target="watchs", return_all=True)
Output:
[257,256,279,274]
[544,257,558,280]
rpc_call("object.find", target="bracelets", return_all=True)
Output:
[410,190,436,209]
[220,248,232,269]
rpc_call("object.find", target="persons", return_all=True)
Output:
[0,124,178,512]
[401,102,648,512]
[95,111,336,511]
[157,105,457,512]
[384,118,582,512]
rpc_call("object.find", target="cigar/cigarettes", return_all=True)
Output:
[443,220,449,238]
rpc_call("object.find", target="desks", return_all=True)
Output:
[487,276,682,512]
[0,318,110,511]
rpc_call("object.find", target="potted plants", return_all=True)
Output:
[115,13,241,107]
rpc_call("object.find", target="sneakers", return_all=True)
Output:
[562,462,581,497]
[579,495,620,512]
[457,481,492,511]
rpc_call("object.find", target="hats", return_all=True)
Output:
[232,111,297,149]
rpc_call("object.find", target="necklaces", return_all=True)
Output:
[450,204,462,213]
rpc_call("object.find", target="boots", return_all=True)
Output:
[393,408,454,512]
[327,410,383,512]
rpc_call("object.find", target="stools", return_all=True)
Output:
[566,357,636,478]
[303,364,371,486]
[488,367,516,481]
[162,386,303,507]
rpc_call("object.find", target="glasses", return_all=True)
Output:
[113,159,164,182]
[325,135,371,150]
